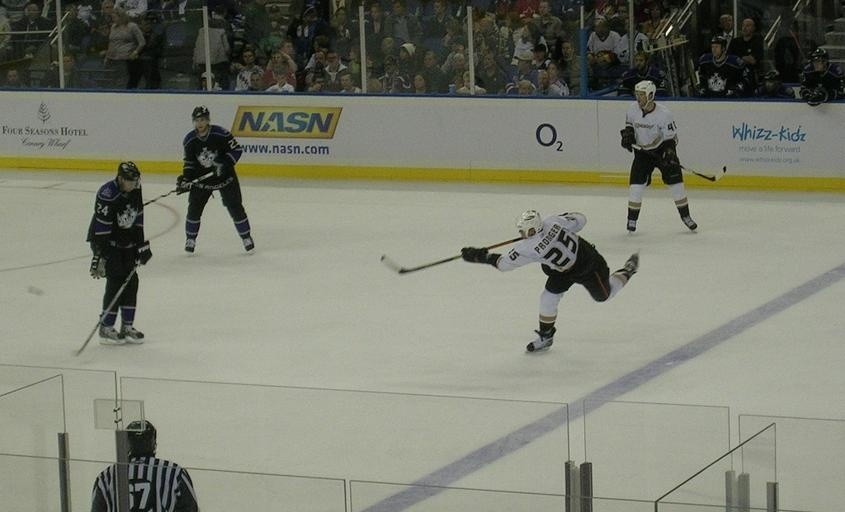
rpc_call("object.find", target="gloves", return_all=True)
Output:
[661,140,679,171]
[808,89,824,106]
[462,247,489,264]
[89,248,109,279]
[174,175,189,195]
[135,241,153,265]
[801,88,826,102]
[213,155,233,178]
[620,127,636,152]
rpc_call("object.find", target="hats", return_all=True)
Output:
[402,42,417,57]
[529,44,546,54]
[269,5,280,13]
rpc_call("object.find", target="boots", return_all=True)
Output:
[122,317,144,339]
[624,259,637,276]
[526,327,556,351]
[99,314,124,341]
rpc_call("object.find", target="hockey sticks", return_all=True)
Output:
[634,144,727,182]
[380,237,526,274]
[72,264,140,356]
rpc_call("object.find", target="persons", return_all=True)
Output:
[88,420,201,511]
[621,80,698,230]
[176,105,254,252]
[84,161,152,341]
[461,208,640,351]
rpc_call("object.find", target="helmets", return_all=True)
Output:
[810,48,831,74]
[710,36,728,51]
[191,105,209,121]
[127,420,157,457]
[634,81,657,103]
[516,210,543,238]
[116,161,141,191]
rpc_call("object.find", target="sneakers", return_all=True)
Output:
[682,216,697,230]
[186,237,196,253]
[242,236,254,250]
[627,217,637,230]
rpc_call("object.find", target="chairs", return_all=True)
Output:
[1,1,845,101]
